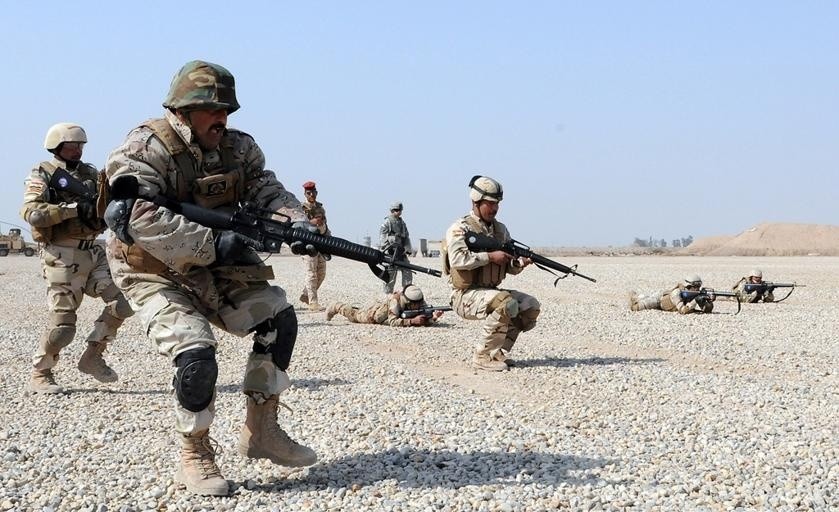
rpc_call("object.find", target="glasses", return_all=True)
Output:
[63,142,84,153]
[686,280,702,286]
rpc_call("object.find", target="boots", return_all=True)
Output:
[77,341,118,383]
[299,295,326,311]
[472,346,516,372]
[239,392,317,467]
[325,302,346,321]
[179,428,229,497]
[31,368,63,394]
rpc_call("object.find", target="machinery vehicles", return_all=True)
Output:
[0,228,34,257]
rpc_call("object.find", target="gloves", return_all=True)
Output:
[287,220,332,262]
[757,287,767,294]
[695,295,709,303]
[77,202,95,221]
[213,230,263,266]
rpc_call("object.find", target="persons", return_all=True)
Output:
[380,200,414,293]
[297,180,329,312]
[323,286,443,329]
[632,274,719,314]
[103,60,330,499]
[445,177,539,371]
[735,271,780,304]
[20,122,133,395]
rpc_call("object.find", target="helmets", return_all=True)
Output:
[391,203,403,210]
[403,285,424,304]
[751,270,762,278]
[162,60,240,116]
[46,122,87,149]
[470,176,503,202]
[683,273,702,288]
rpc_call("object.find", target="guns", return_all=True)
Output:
[400,307,453,319]
[463,232,597,284]
[176,201,444,279]
[49,166,99,205]
[744,283,807,293]
[679,288,743,303]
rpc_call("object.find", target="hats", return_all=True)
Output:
[304,182,315,190]
